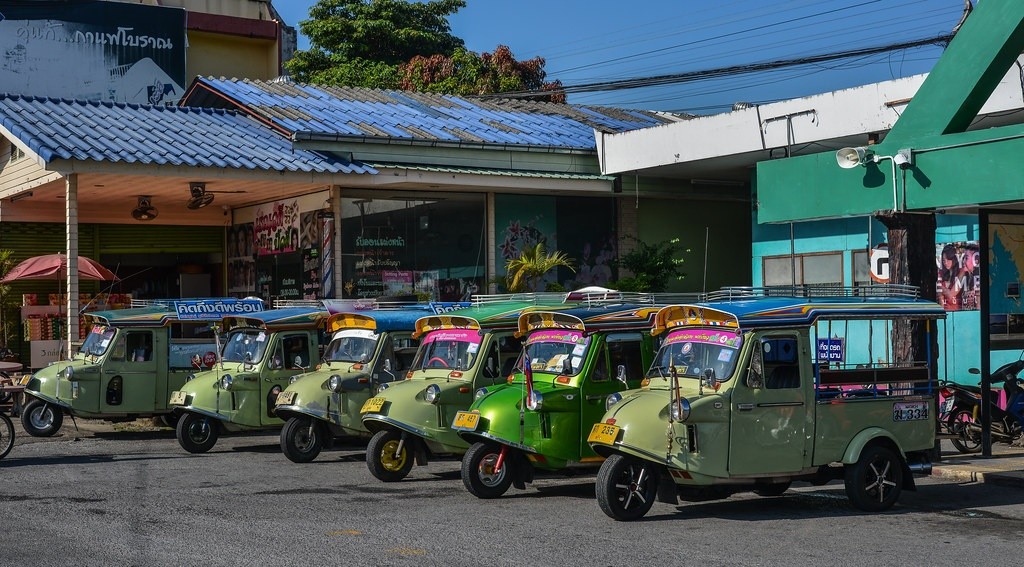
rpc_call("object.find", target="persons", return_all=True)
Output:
[935,242,981,311]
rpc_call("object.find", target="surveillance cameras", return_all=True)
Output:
[894,154,910,169]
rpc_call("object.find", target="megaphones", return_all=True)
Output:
[837,146,866,168]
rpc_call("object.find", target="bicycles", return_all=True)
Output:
[0,410,16,462]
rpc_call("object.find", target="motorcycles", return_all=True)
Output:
[359,298,572,486]
[587,280,946,523]
[275,308,441,466]
[18,303,261,438]
[167,305,336,455]
[449,291,699,498]
[937,350,1024,454]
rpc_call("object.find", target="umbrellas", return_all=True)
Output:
[0,250,121,284]
